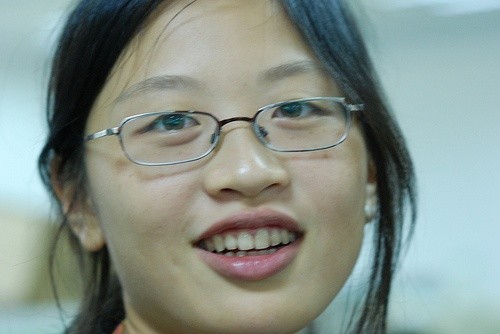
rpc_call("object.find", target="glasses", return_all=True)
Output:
[79,94,367,169]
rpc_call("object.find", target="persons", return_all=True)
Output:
[33,0,426,334]
